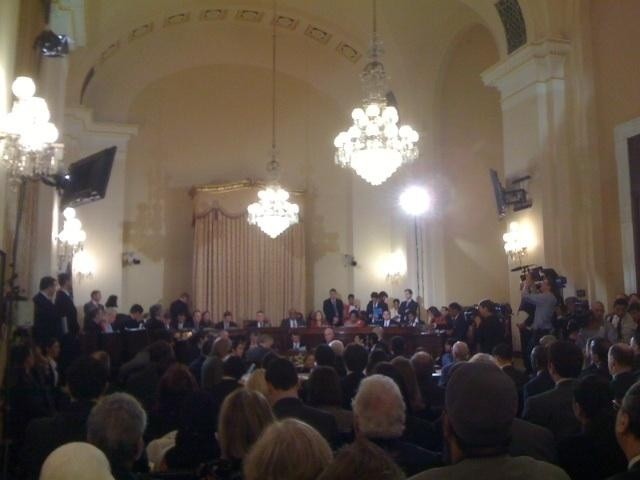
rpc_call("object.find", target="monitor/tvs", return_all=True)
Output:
[489,166,531,218]
[54,143,118,215]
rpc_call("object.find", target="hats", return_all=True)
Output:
[446,360,518,446]
[362,361,401,381]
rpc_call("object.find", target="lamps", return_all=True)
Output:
[502,218,537,267]
[334,0,420,187]
[55,206,87,272]
[0,77,66,193]
[247,0,301,239]
[374,248,409,286]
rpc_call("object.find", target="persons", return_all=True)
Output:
[1,268,640,480]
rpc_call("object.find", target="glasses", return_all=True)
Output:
[610,397,632,415]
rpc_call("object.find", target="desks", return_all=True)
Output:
[93,326,446,357]
